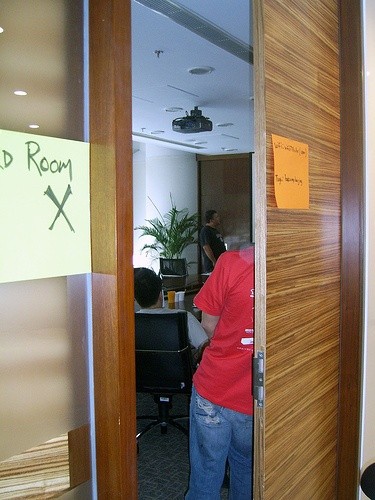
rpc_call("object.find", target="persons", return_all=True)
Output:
[185,246,255,500]
[133,268,209,410]
[200,210,225,274]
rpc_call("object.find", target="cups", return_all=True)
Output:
[167,291,175,303]
[175,293,179,302]
[177,292,185,301]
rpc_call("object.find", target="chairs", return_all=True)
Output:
[135,311,201,453]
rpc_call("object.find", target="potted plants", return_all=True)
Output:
[134,207,206,275]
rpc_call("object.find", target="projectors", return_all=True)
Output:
[172,115,213,133]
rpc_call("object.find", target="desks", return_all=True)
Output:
[160,273,209,321]
